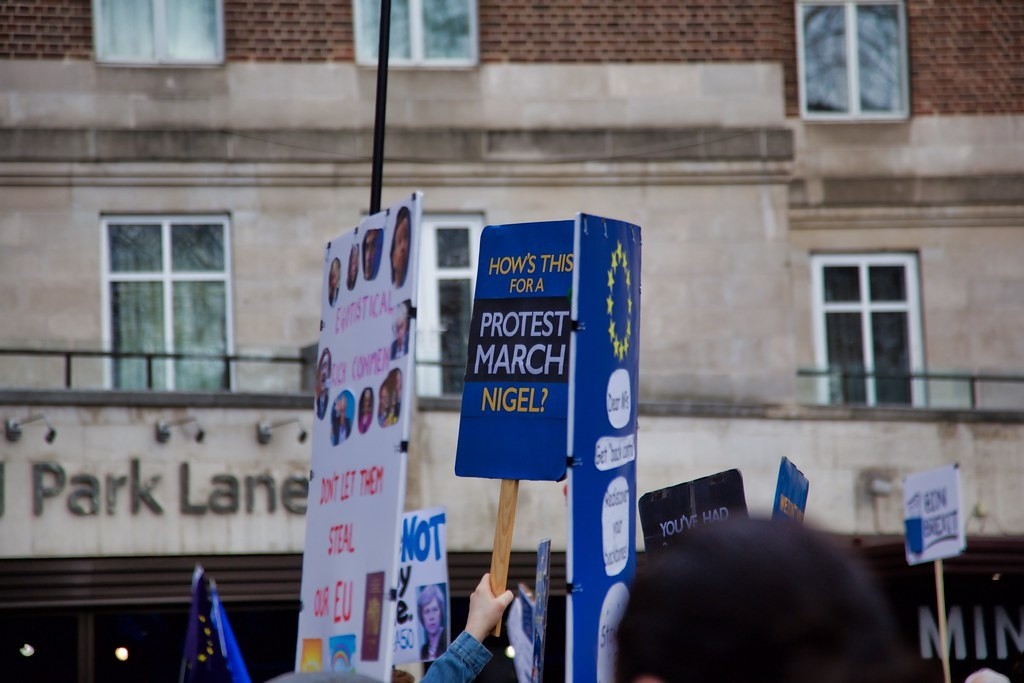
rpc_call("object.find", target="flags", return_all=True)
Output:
[179,570,252,683]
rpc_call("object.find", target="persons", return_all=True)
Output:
[377,370,402,427]
[328,398,352,444]
[327,260,341,306]
[361,229,379,280]
[390,302,409,359]
[316,353,331,419]
[274,514,1011,683]
[347,245,358,291]
[390,211,411,286]
[357,390,375,434]
[414,585,447,660]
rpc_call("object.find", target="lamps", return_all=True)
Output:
[256,417,307,445]
[153,415,205,444]
[4,413,57,444]
[869,478,893,498]
